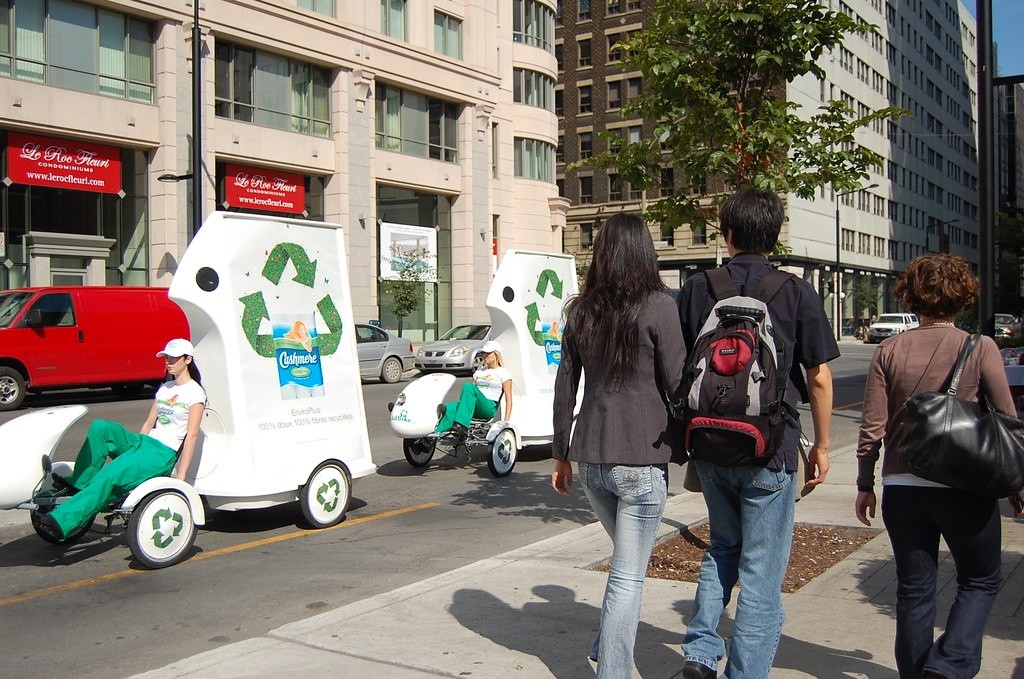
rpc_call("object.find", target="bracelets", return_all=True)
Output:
[858,475,874,491]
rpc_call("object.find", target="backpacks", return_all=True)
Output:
[680,266,809,466]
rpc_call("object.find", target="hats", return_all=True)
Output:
[479,341,502,353]
[156,339,194,357]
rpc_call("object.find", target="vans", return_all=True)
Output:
[0,285,191,413]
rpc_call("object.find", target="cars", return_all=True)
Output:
[353,323,416,384]
[976,313,1022,342]
[414,322,492,376]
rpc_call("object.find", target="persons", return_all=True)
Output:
[435,341,512,438]
[676,187,841,679]
[855,254,1024,679]
[552,213,688,679]
[33,337,207,543]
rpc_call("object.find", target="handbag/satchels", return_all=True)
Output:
[901,332,1024,499]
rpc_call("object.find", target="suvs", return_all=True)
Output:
[867,313,920,343]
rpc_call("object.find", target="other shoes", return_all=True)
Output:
[451,427,468,441]
[682,660,717,679]
[32,510,61,537]
[52,473,73,493]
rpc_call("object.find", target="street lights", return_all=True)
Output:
[925,218,962,254]
[835,182,881,341]
[156,0,203,238]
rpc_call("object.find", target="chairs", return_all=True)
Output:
[469,389,504,439]
